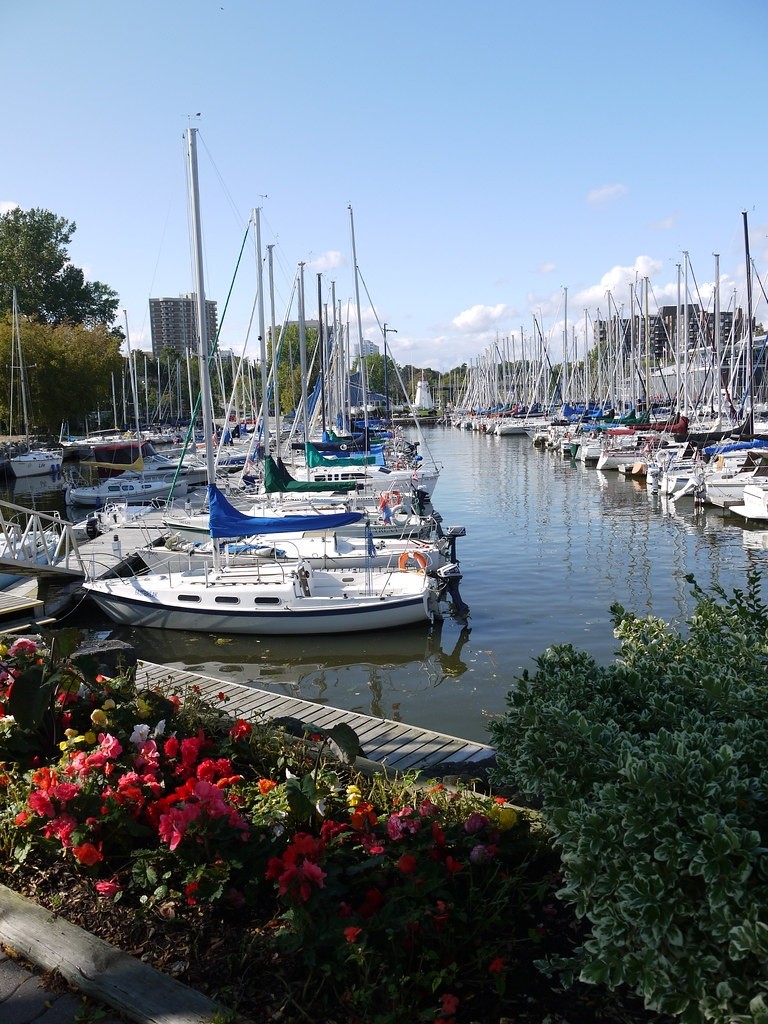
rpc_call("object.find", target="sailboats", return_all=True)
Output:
[1,192,767,634]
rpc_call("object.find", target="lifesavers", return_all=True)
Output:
[399,553,425,574]
[380,490,401,510]
[657,450,669,463]
[391,505,411,525]
